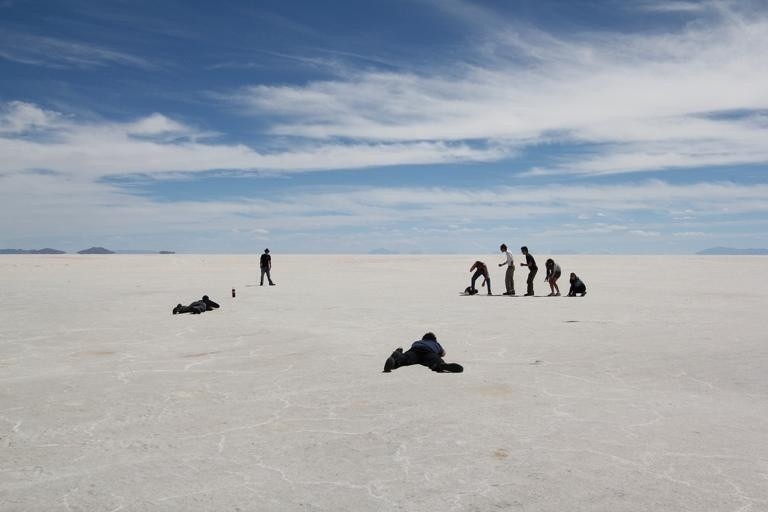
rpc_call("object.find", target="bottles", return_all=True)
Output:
[232,288,235,298]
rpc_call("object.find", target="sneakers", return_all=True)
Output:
[524,291,534,296]
[439,363,462,373]
[192,307,200,314]
[172,304,181,314]
[503,289,514,295]
[570,292,586,296]
[384,350,400,371]
[548,292,560,296]
[260,283,275,285]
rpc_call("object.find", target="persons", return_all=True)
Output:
[383,331,464,374]
[519,245,538,296]
[258,248,276,286]
[545,259,562,297]
[469,260,493,296]
[497,243,516,296]
[172,294,219,315]
[565,273,588,297]
